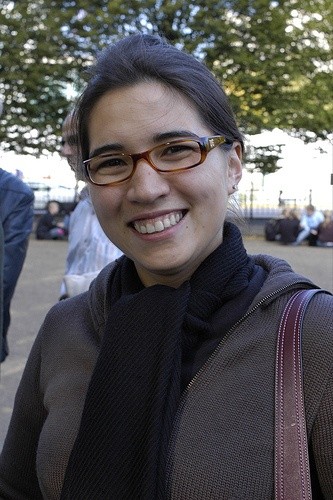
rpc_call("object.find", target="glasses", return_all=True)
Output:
[82,135,228,186]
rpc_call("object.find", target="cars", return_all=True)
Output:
[265,216,320,246]
[36,208,74,240]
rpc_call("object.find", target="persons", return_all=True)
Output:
[265,205,333,251]
[1,169,35,367]
[0,35,333,500]
[37,199,67,240]
[57,106,125,307]
